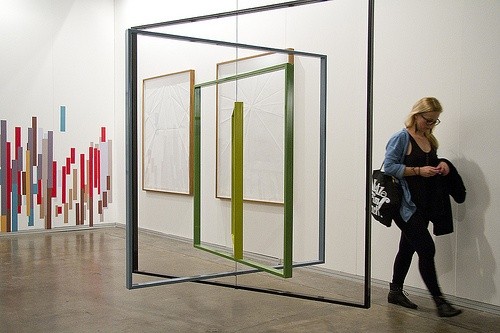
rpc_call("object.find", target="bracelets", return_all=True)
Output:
[412,166,420,176]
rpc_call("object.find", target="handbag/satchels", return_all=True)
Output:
[371,169,403,227]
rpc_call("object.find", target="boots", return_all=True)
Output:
[387,282,419,308]
[433,297,462,318]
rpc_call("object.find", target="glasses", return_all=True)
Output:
[420,112,441,126]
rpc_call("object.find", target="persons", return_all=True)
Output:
[383,96,463,318]
